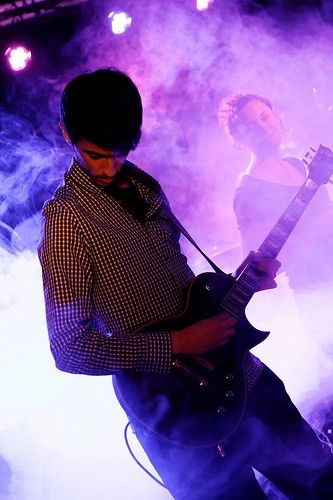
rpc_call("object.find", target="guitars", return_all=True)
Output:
[111,145,333,446]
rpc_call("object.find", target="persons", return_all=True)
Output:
[37,66,333,500]
[218,93,333,359]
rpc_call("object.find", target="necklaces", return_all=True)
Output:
[262,162,298,185]
